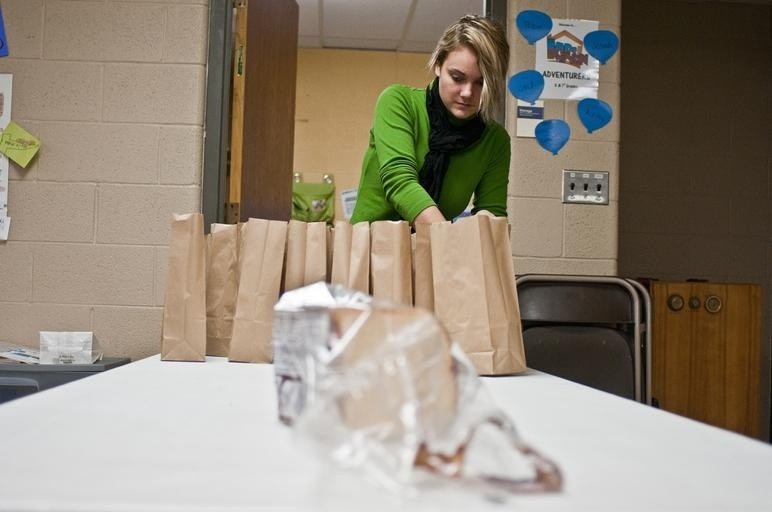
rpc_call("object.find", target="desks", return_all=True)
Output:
[638,279,763,439]
[0,352,768,512]
[2,356,129,392]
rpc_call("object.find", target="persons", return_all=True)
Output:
[349,13,510,232]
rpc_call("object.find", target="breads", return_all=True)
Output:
[329,307,458,439]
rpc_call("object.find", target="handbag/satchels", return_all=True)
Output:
[162,213,526,377]
